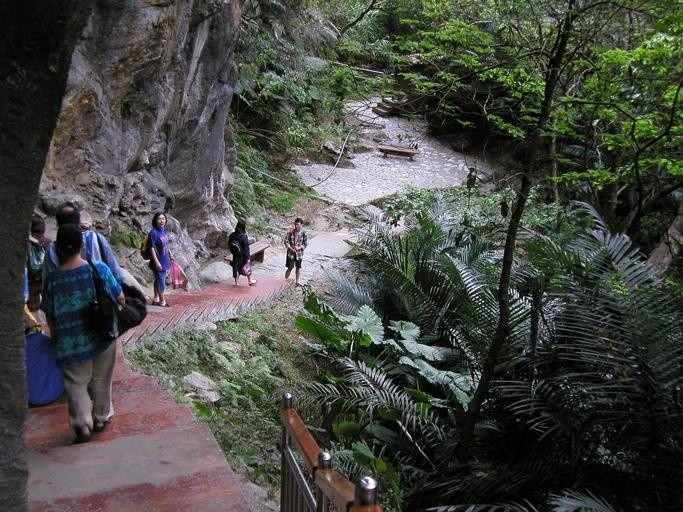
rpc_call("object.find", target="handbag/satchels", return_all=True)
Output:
[141,234,151,260]
[91,292,114,331]
[117,283,146,326]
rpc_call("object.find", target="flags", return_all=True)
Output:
[171,261,189,292]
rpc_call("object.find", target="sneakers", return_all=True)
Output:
[248,280,256,285]
[152,301,169,307]
[76,420,106,442]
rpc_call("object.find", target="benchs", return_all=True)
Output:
[376,144,420,161]
[223,241,270,267]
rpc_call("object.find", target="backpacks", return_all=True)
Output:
[230,240,242,255]
[28,241,46,283]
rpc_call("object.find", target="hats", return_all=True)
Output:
[56,223,80,248]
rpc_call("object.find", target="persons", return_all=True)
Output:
[145,212,174,309]
[228,217,257,286]
[39,224,125,445]
[25,212,51,326]
[42,202,124,296]
[284,218,308,286]
